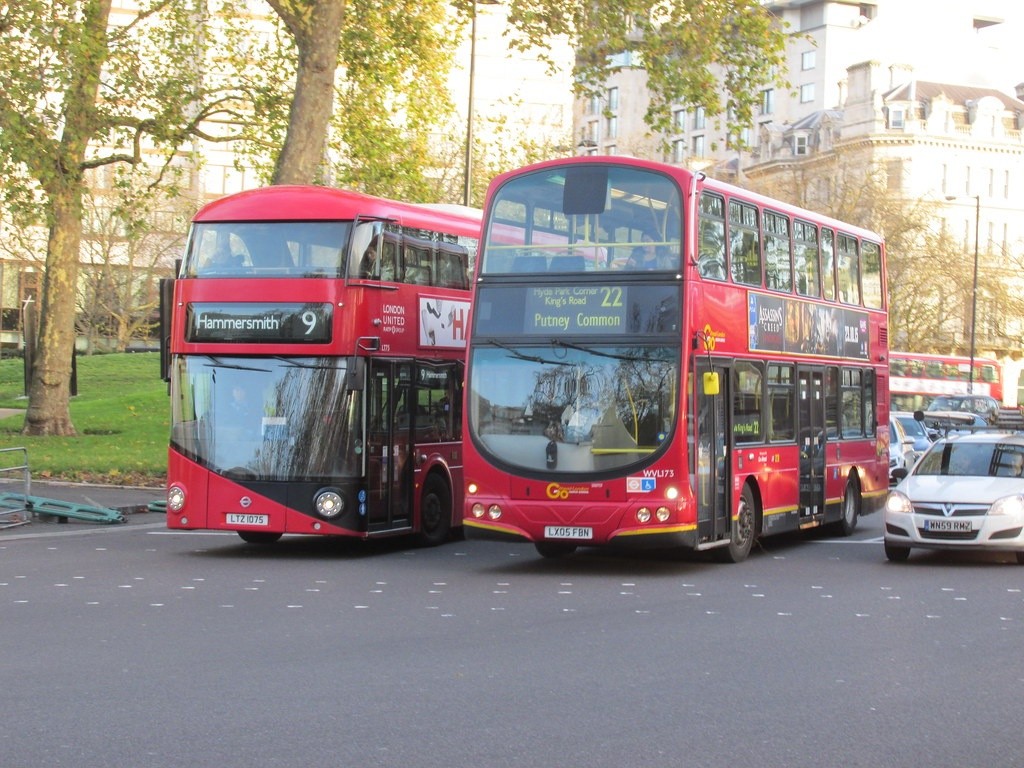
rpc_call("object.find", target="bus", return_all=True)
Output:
[888,351,1001,414]
[463,156,888,560]
[163,184,614,546]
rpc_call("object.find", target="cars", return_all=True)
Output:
[927,411,991,438]
[890,412,937,463]
[927,395,1001,423]
[882,431,1024,569]
[889,417,916,488]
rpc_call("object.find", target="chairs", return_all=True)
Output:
[732,393,873,441]
[367,404,463,443]
[511,252,808,297]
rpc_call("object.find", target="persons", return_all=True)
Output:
[223,383,266,425]
[357,244,380,281]
[999,453,1024,478]
[624,228,673,269]
[203,241,245,268]
[549,369,615,445]
